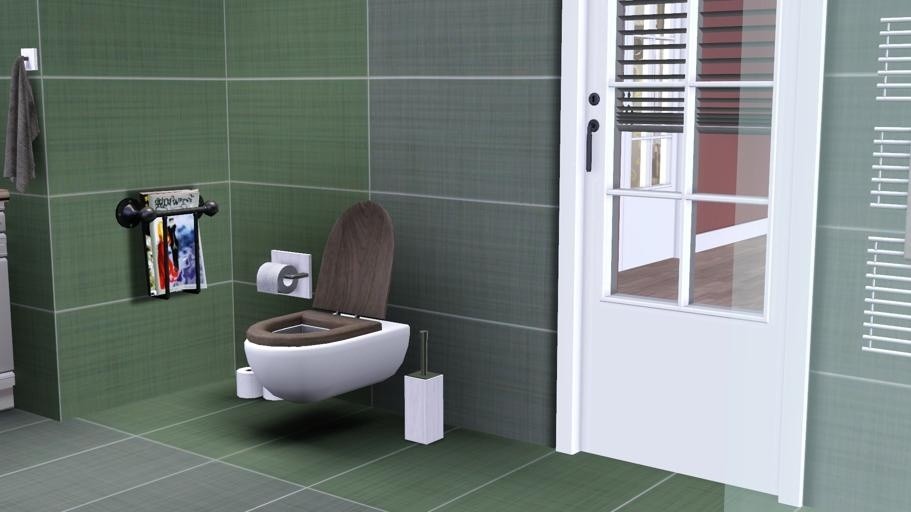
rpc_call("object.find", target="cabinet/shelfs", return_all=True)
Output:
[0,198,16,412]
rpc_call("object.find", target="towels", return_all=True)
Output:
[4,56,40,191]
